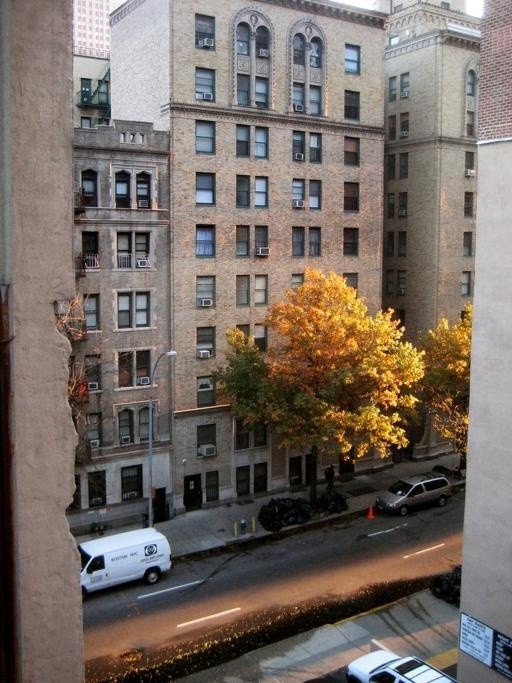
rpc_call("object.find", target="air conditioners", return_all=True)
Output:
[139,200,148,208]
[203,38,215,47]
[258,49,268,58]
[198,350,210,358]
[128,492,135,498]
[396,288,405,295]
[294,104,303,112]
[401,92,408,97]
[88,382,98,390]
[90,497,102,505]
[137,260,151,268]
[204,93,213,100]
[466,169,474,178]
[90,440,100,449]
[201,298,212,306]
[292,200,304,208]
[293,153,303,160]
[256,247,270,256]
[399,210,407,216]
[201,444,216,457]
[400,131,408,137]
[121,435,130,444]
[138,377,150,385]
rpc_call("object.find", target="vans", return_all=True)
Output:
[81,525,173,598]
[374,473,452,517]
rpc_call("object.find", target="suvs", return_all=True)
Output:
[344,647,461,683]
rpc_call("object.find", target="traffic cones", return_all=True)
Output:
[366,503,375,520]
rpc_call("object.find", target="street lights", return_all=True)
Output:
[146,349,177,529]
